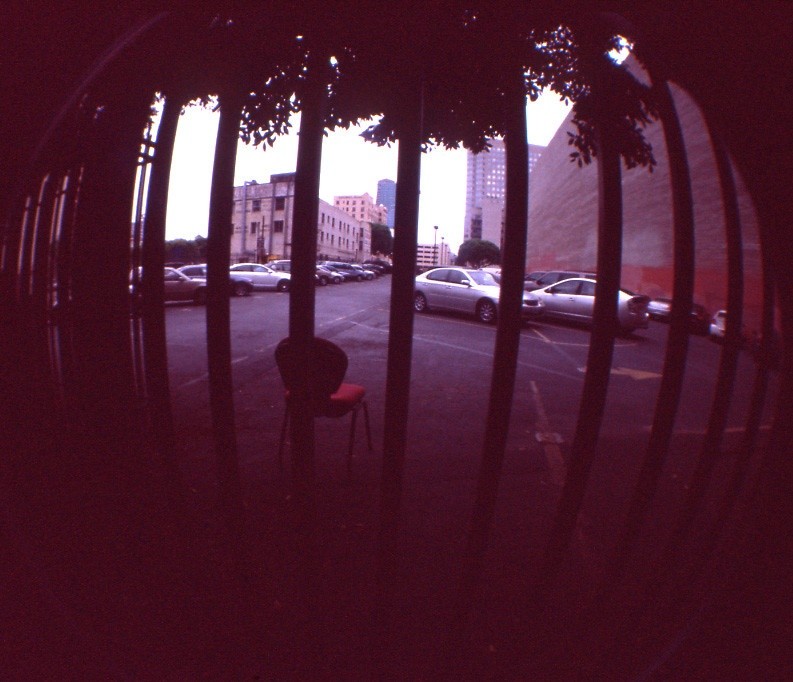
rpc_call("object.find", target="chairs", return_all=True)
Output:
[275,337,371,485]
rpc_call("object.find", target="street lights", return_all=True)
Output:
[433,225,439,265]
[441,236,444,266]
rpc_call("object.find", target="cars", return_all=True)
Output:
[688,303,711,330]
[362,263,384,277]
[646,296,674,319]
[351,265,375,281]
[479,267,598,292]
[258,259,320,286]
[363,258,393,274]
[418,267,544,322]
[315,265,345,286]
[530,276,650,333]
[707,310,728,338]
[326,262,366,284]
[130,265,209,306]
[171,265,254,296]
[323,265,350,281]
[230,262,291,292]
